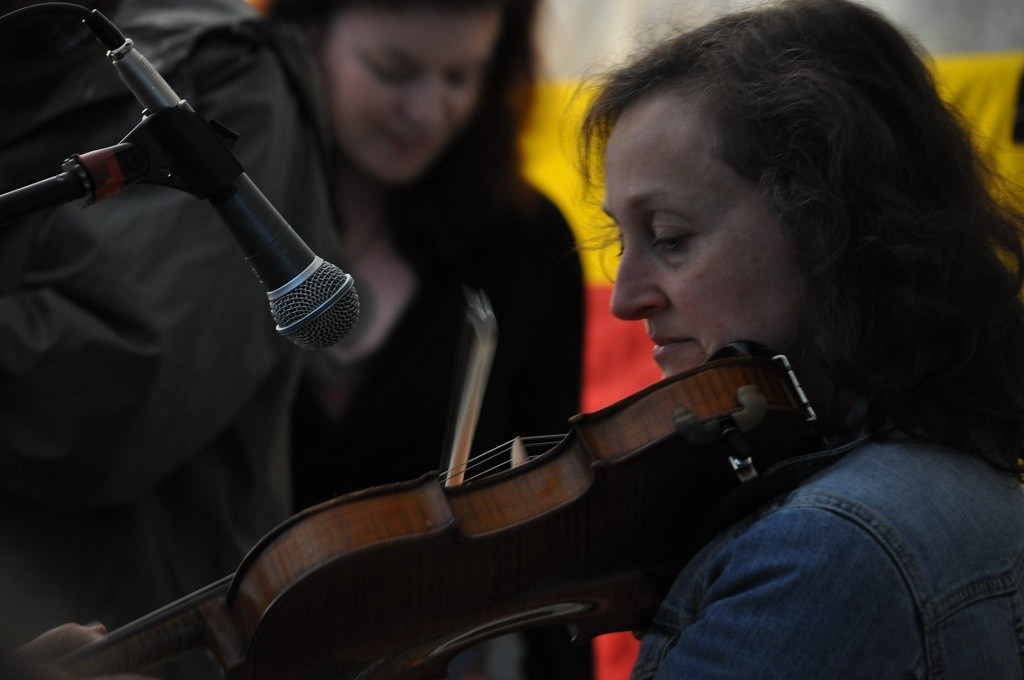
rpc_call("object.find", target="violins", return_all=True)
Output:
[40,339,835,678]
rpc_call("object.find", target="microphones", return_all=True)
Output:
[83,10,361,352]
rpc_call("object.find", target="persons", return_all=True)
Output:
[0,1,332,680]
[0,0,1024,680]
[289,0,596,680]
